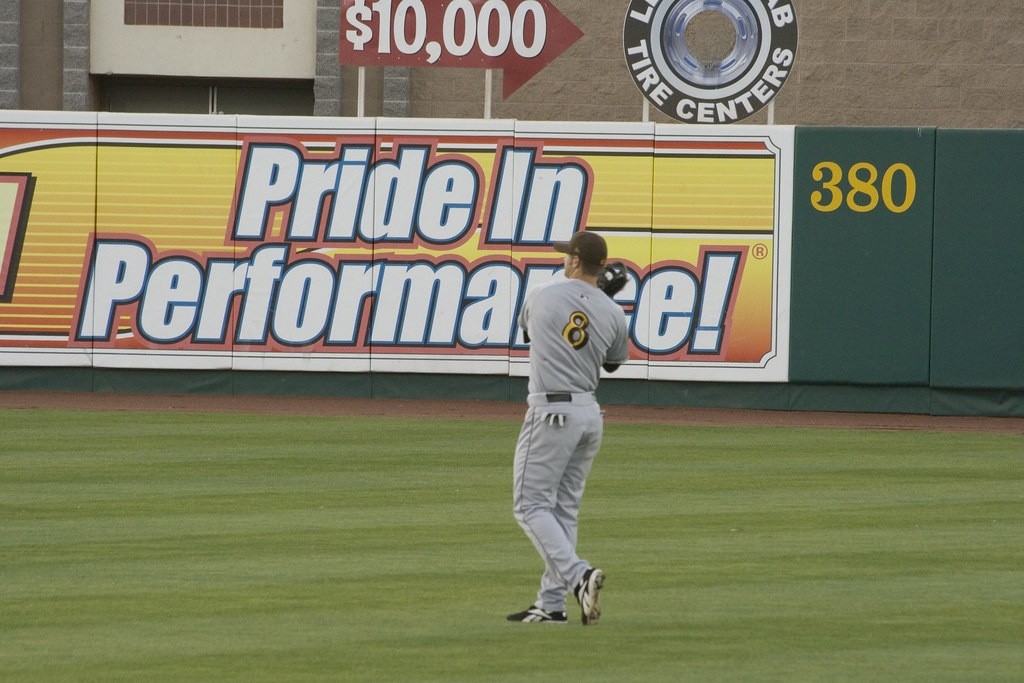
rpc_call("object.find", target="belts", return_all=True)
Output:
[549,394,572,403]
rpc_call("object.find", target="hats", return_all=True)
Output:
[552,229,608,266]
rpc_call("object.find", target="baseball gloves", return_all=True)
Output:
[596,261,629,299]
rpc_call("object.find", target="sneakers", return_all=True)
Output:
[574,567,606,625]
[506,606,570,626]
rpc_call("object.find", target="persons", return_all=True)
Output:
[505,231,629,624]
